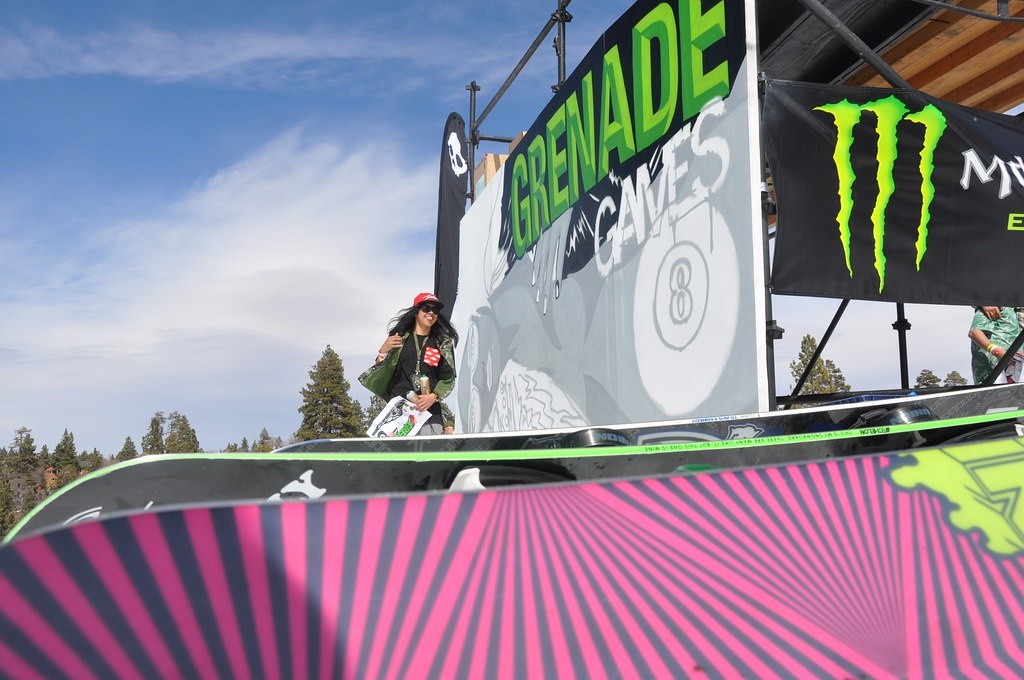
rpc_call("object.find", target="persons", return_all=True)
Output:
[968,304,1024,385]
[359,292,459,436]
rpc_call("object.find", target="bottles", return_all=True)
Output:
[406,391,418,402]
[420,374,430,395]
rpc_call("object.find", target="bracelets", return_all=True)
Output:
[377,348,388,357]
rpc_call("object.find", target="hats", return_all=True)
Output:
[413,293,445,311]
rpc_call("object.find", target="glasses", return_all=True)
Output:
[419,304,440,315]
